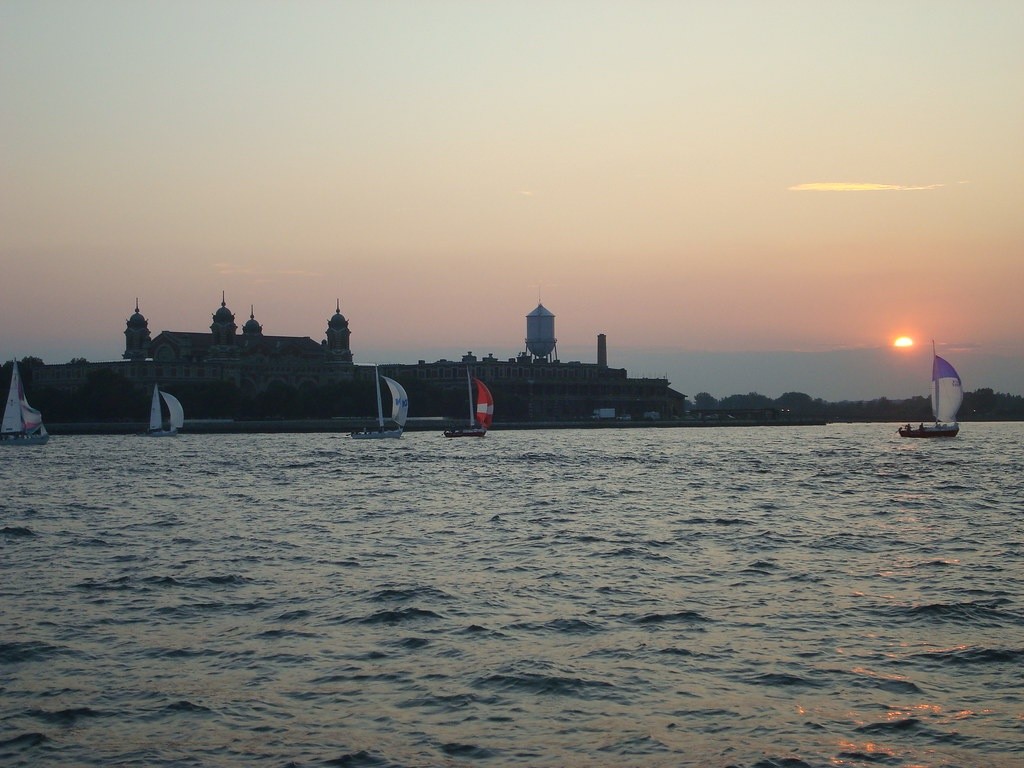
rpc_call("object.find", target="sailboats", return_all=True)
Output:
[146,381,184,436]
[893,339,965,436]
[0,357,49,446]
[442,362,494,438]
[345,362,411,439]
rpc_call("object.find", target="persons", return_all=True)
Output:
[905,424,911,432]
[444,424,456,433]
[919,423,924,430]
